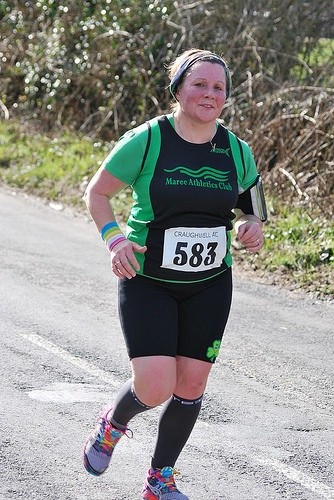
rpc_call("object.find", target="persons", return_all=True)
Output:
[83,48,268,500]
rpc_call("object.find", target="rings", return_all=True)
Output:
[114,261,121,265]
[257,240,261,245]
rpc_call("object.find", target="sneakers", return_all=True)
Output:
[84,405,133,475]
[141,467,188,500]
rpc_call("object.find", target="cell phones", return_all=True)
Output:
[256,176,267,221]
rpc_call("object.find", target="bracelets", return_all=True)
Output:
[98,221,130,253]
